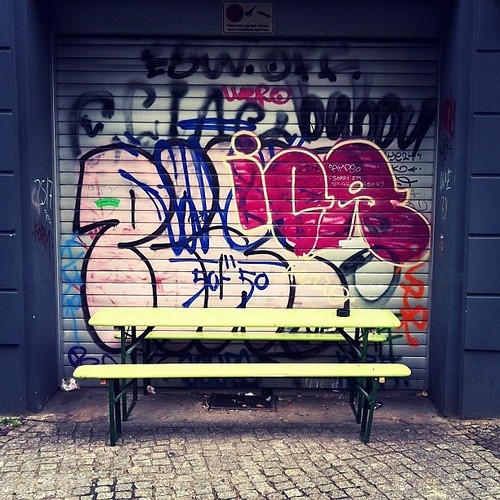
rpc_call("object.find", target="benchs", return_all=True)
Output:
[73,362,412,447]
[113,331,387,397]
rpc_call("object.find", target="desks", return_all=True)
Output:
[86,306,402,424]
